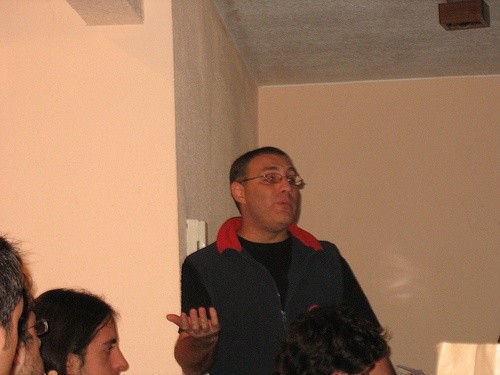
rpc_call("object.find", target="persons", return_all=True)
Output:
[276,306,388,374]
[0,236,26,375]
[30,289,130,375]
[9,290,46,375]
[165,146,399,375]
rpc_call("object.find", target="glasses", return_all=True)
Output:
[23,318,48,335]
[239,173,302,186]
